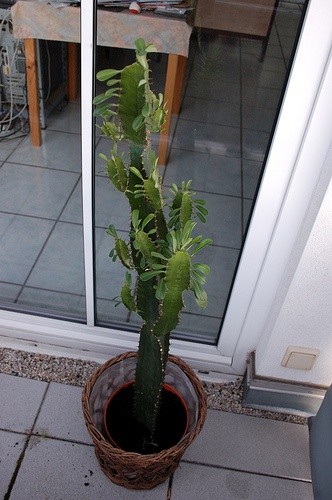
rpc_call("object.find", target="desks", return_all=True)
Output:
[11,0,197,166]
[194,0,278,62]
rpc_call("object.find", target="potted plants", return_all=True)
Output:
[82,38,213,491]
